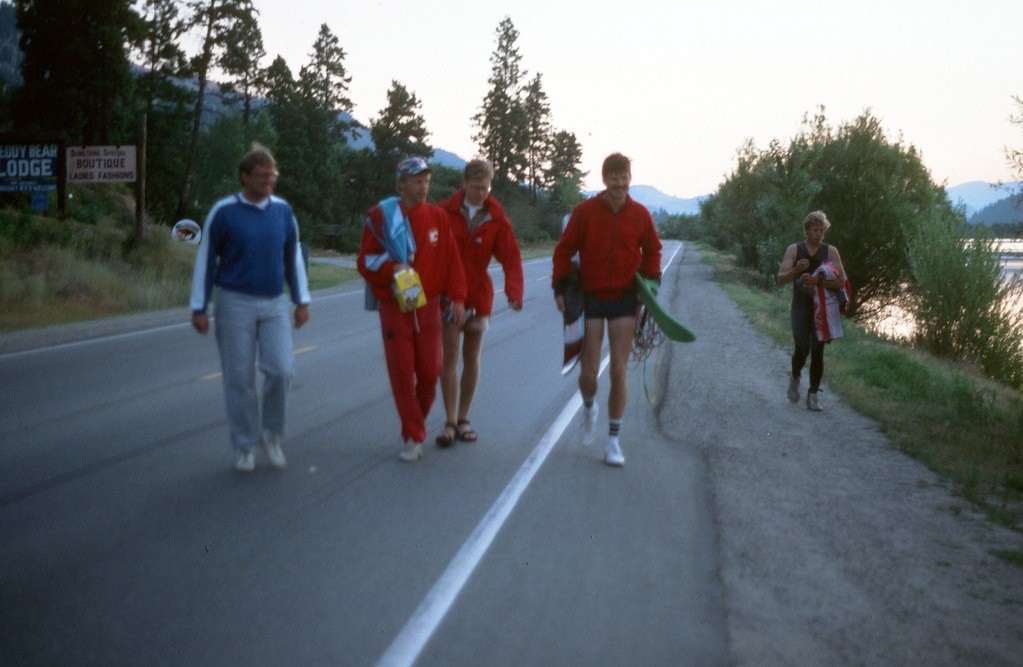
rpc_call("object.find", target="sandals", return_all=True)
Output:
[436,423,457,446]
[457,420,478,442]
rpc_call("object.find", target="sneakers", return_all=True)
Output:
[399,438,423,462]
[581,405,598,445]
[604,436,625,465]
[787,374,800,403]
[237,449,256,471]
[807,390,823,411]
[261,431,286,471]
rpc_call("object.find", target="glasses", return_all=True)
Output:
[248,171,278,181]
[467,183,491,192]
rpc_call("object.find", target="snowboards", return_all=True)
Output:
[561,213,586,377]
[636,272,696,343]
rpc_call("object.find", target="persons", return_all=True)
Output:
[190,151,311,471]
[778,212,847,410]
[437,159,524,445]
[357,158,467,461]
[553,153,663,467]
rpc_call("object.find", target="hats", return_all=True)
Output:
[396,157,434,180]
